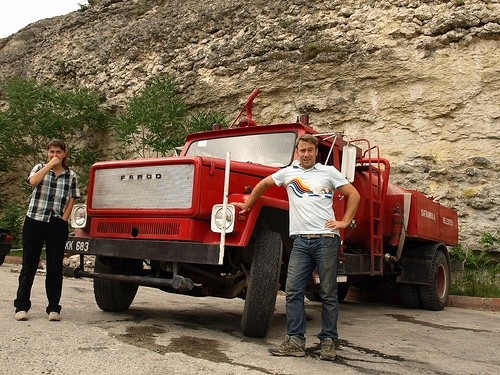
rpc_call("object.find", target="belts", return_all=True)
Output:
[296,233,337,238]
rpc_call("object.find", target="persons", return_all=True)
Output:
[231,134,360,359]
[15,139,81,321]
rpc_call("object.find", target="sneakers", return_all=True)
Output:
[267,335,305,357]
[320,337,337,361]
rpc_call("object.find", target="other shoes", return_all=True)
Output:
[15,310,28,321]
[48,312,60,321]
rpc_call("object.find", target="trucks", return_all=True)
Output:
[62,85,460,341]
